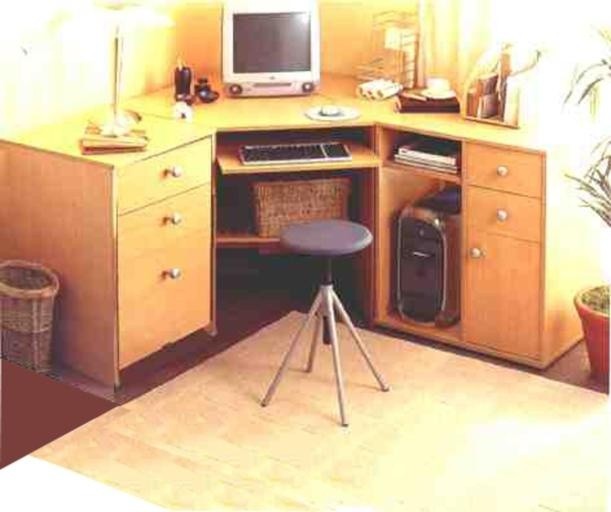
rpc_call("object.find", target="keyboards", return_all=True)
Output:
[238,142,353,166]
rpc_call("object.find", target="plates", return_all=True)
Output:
[305,105,364,121]
[422,89,456,100]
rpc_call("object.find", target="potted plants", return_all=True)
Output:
[559,24,611,382]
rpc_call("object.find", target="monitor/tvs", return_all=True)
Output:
[221,0,320,97]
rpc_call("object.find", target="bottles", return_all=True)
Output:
[194,78,210,95]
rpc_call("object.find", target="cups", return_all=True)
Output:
[175,66,191,93]
[427,78,449,97]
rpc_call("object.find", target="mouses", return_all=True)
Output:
[319,105,339,116]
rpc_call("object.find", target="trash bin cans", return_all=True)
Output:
[0,259,58,370]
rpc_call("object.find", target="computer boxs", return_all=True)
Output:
[397,185,462,329]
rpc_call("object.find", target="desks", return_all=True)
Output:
[0,73,599,389]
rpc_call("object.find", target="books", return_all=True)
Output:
[394,138,460,174]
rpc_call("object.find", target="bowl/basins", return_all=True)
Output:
[175,91,219,105]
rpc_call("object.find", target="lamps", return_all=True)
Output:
[70,0,176,136]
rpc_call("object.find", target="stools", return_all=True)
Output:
[259,220,389,426]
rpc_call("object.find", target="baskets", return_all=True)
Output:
[247,179,351,254]
